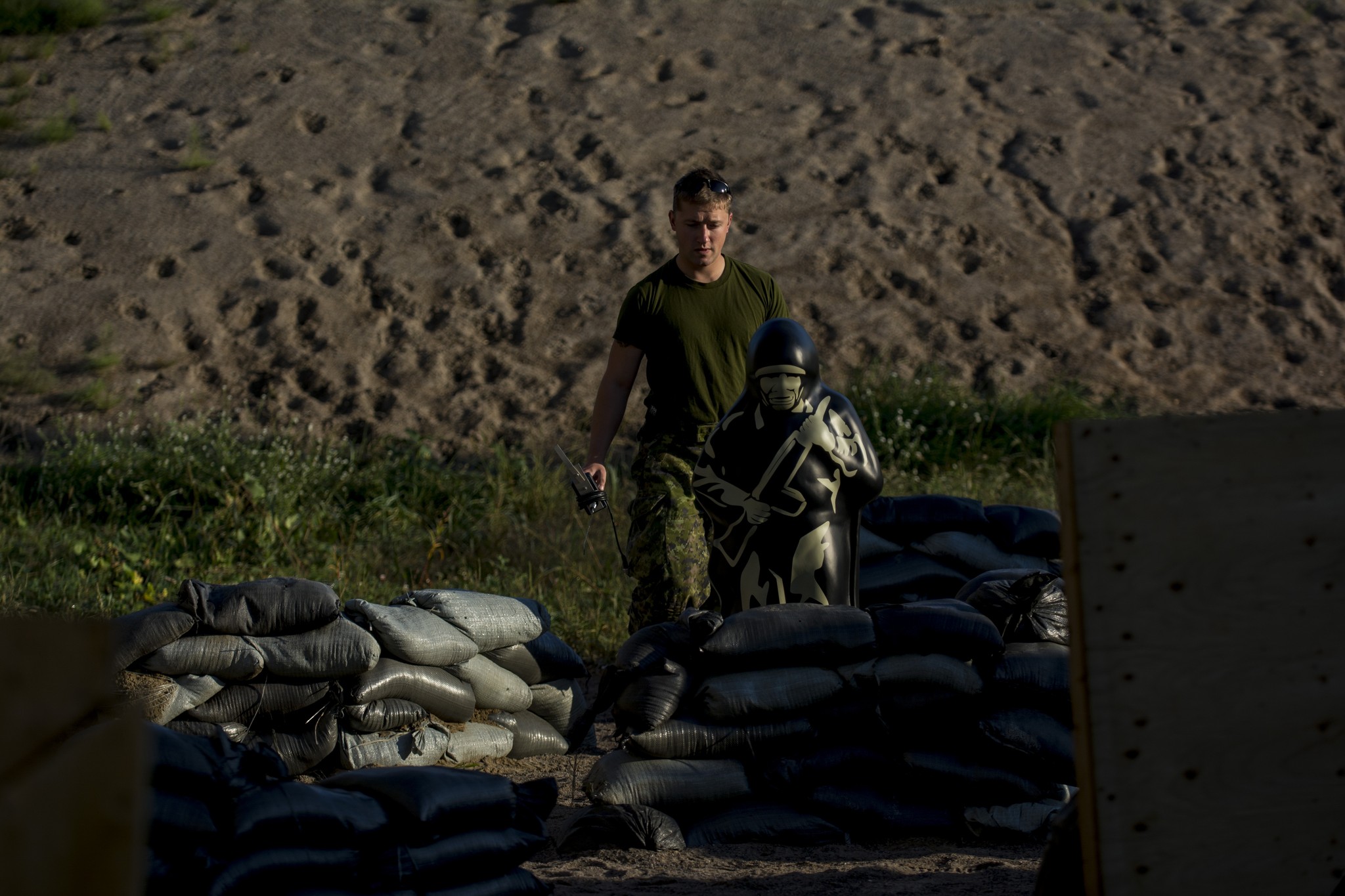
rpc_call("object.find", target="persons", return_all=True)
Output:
[692,317,883,608]
[578,168,792,633]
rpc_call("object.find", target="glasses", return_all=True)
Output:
[675,175,731,193]
